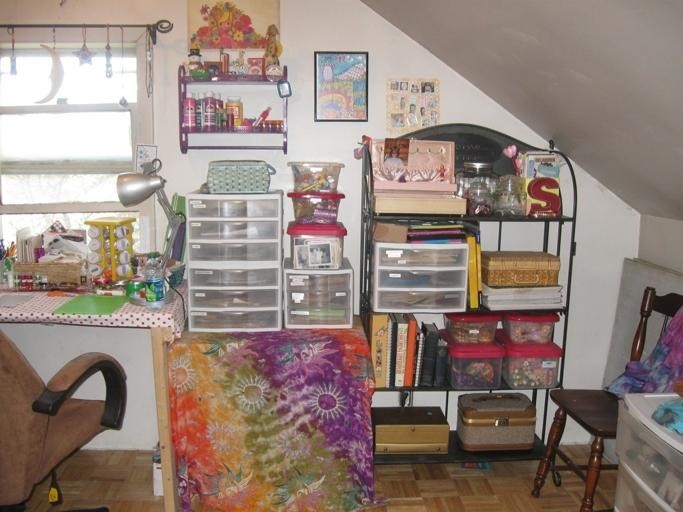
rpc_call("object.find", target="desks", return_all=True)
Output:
[167,315,376,512]
[0,279,188,511]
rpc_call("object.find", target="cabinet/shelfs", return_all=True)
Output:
[178,65,288,155]
[186,190,281,333]
[613,393,683,512]
[362,123,576,486]
[370,242,469,314]
[283,256,353,329]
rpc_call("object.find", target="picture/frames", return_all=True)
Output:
[314,51,369,122]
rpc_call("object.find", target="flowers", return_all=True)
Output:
[503,144,526,176]
[191,2,267,48]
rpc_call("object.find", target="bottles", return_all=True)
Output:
[261,119,285,131]
[143,252,165,304]
[455,161,527,217]
[184,91,244,128]
[186,48,202,76]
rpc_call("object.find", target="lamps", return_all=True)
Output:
[116,158,185,271]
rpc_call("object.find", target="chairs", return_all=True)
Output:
[531,287,683,512]
[0,329,127,511]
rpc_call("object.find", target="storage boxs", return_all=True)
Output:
[287,221,347,270]
[289,162,344,194]
[496,329,562,388]
[438,329,506,389]
[443,313,503,344]
[287,194,345,225]
[371,407,450,454]
[503,313,559,344]
